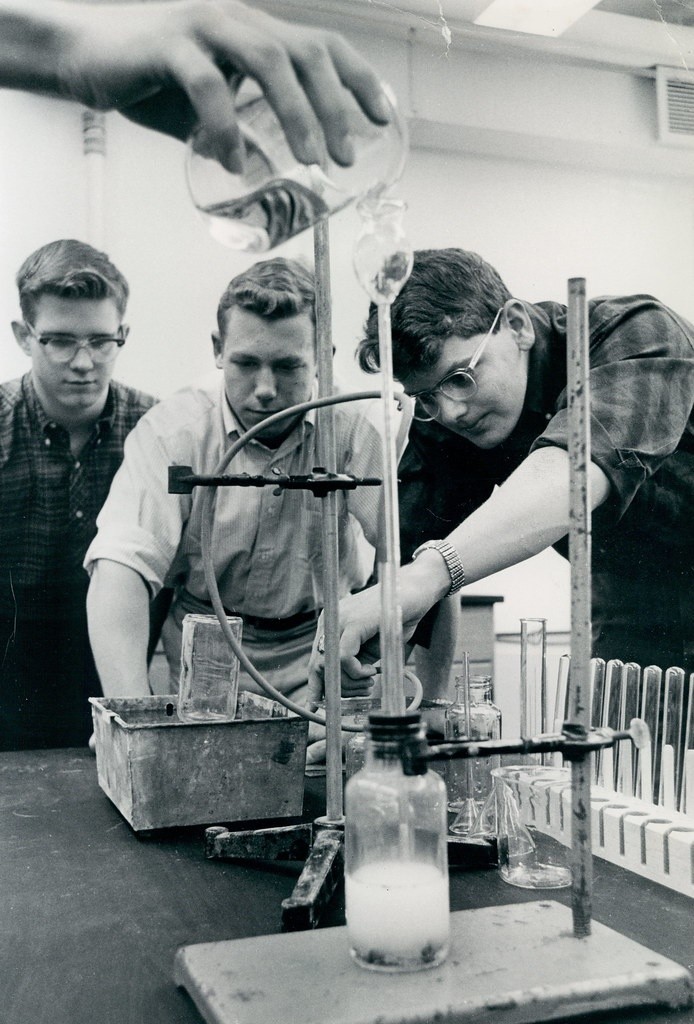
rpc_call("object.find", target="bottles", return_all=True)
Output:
[346,704,382,779]
[344,712,452,972]
[445,676,502,812]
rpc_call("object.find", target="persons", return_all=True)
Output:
[1,239,163,753]
[83,257,462,751]
[304,248,694,802]
[0,0,394,176]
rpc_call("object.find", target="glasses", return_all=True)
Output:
[397,307,505,421]
[25,320,124,363]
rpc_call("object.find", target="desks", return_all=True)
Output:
[0,747,694,1024]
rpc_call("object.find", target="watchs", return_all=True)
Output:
[412,539,465,607]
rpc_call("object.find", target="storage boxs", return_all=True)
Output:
[88,690,310,840]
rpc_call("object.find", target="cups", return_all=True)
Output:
[185,75,410,255]
[491,766,572,889]
[177,615,244,722]
